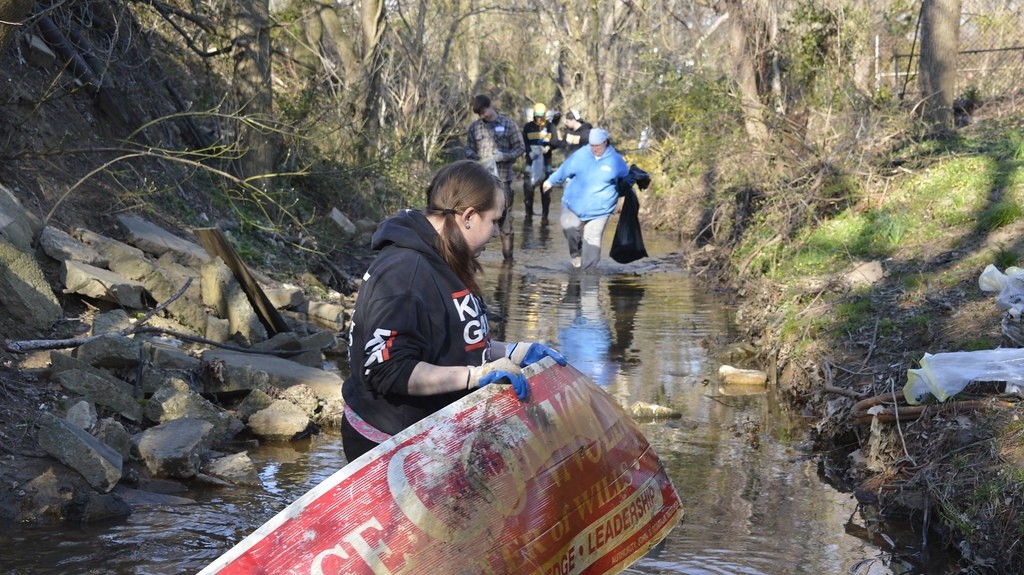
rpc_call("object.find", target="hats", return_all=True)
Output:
[589,128,608,145]
[566,112,584,123]
[533,103,546,118]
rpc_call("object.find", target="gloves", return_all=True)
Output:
[465,357,529,400]
[508,341,567,368]
[529,152,539,160]
[543,180,551,193]
[542,146,550,154]
[492,151,502,162]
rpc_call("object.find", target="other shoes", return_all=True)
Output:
[574,256,581,267]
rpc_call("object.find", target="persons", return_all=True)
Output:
[543,128,629,273]
[523,102,558,225]
[463,94,525,262]
[556,110,593,184]
[524,106,562,179]
[341,157,567,466]
